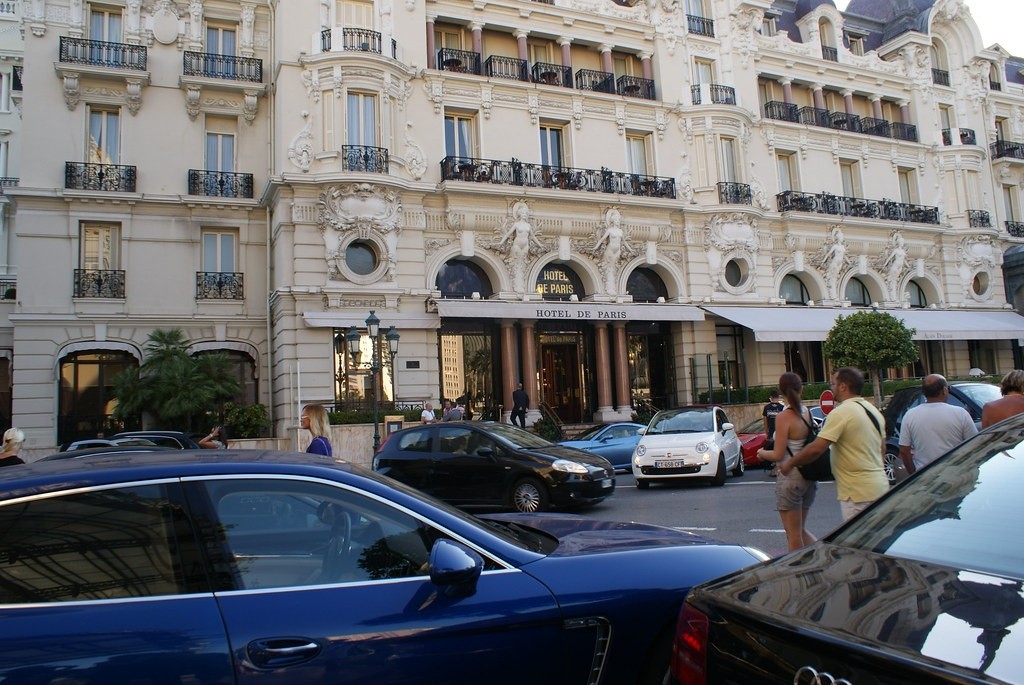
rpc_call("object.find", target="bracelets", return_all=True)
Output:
[210,434,212,438]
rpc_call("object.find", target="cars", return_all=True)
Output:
[373,420,617,517]
[671,410,1024,685]
[631,408,744,489]
[881,381,1005,485]
[736,405,826,470]
[0,457,772,685]
[555,421,661,473]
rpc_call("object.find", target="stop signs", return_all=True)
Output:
[820,390,835,415]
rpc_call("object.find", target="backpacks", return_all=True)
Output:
[786,407,836,482]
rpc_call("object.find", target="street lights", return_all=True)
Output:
[334,331,352,408]
[345,309,401,455]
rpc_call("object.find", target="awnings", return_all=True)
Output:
[431,299,706,321]
[701,306,1024,341]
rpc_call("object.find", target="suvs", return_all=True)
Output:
[31,430,200,461]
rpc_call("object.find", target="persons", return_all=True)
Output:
[816,228,854,300]
[981,369,1024,430]
[300,404,332,457]
[421,401,473,425]
[453,437,469,454]
[756,373,830,553]
[198,425,229,449]
[0,427,26,467]
[762,392,784,477]
[899,374,979,476]
[97,431,104,439]
[498,200,541,293]
[510,383,529,429]
[879,234,912,302]
[779,367,890,521]
[593,209,639,296]
